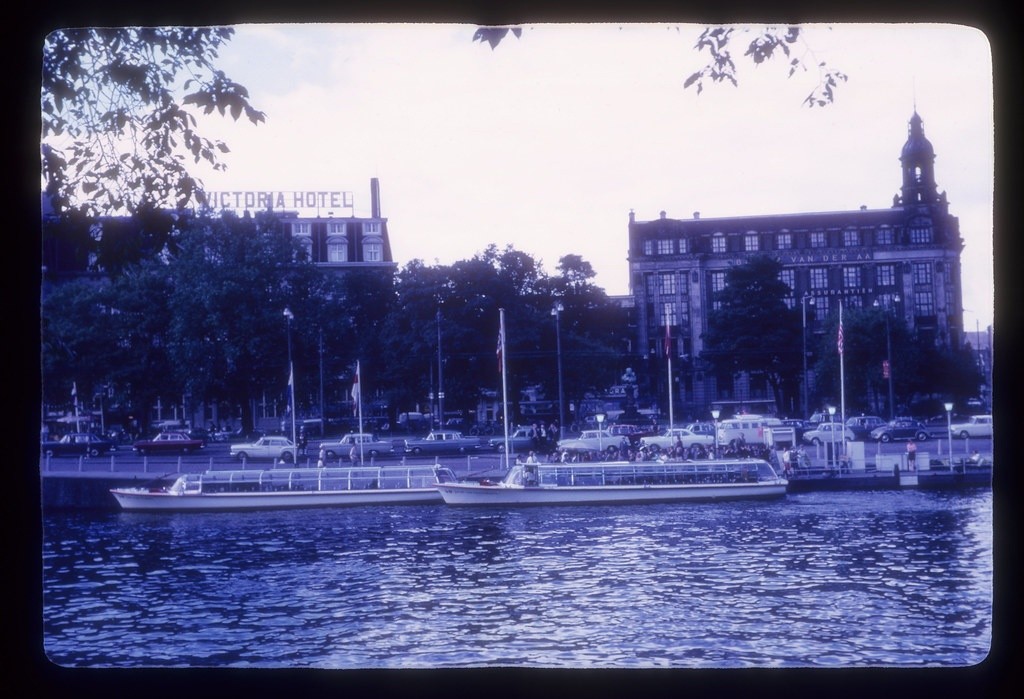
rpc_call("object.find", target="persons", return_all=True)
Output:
[210,422,217,444]
[297,432,307,456]
[529,425,559,452]
[49,426,141,445]
[348,444,359,478]
[651,419,660,435]
[516,435,811,485]
[906,439,917,472]
[965,450,980,464]
[381,421,390,435]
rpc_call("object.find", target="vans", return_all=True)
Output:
[717,415,783,450]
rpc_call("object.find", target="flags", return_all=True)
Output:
[664,314,671,357]
[286,370,293,417]
[71,386,78,407]
[351,365,359,417]
[837,308,844,356]
[497,318,503,372]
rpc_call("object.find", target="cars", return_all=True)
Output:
[230,428,551,464]
[846,416,886,441]
[802,423,855,447]
[950,415,993,439]
[869,418,930,443]
[782,414,841,445]
[133,420,242,456]
[42,432,119,458]
[555,423,716,455]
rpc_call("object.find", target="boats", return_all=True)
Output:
[109,464,459,512]
[431,459,789,509]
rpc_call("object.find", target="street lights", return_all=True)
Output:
[283,307,293,442]
[828,407,836,467]
[551,301,565,440]
[595,414,606,453]
[944,403,954,472]
[711,411,720,453]
[873,292,901,420]
[801,296,815,419]
